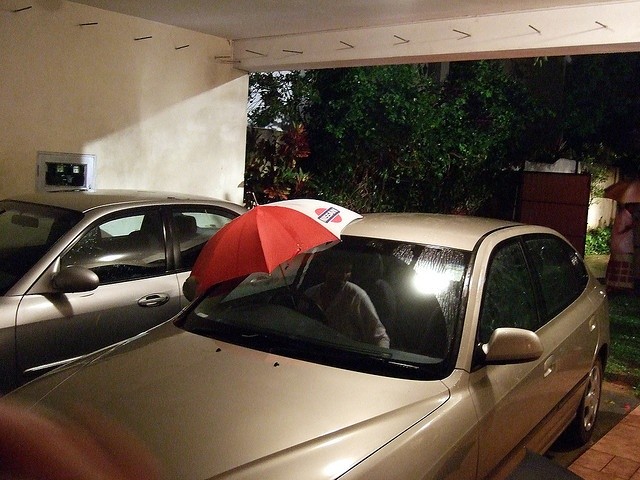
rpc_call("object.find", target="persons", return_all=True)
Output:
[299,263,390,348]
[605,203,638,297]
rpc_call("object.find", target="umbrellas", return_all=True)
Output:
[602,177,640,204]
[187,192,363,311]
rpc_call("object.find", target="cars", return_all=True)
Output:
[0,213,610,480]
[1,191,249,399]
